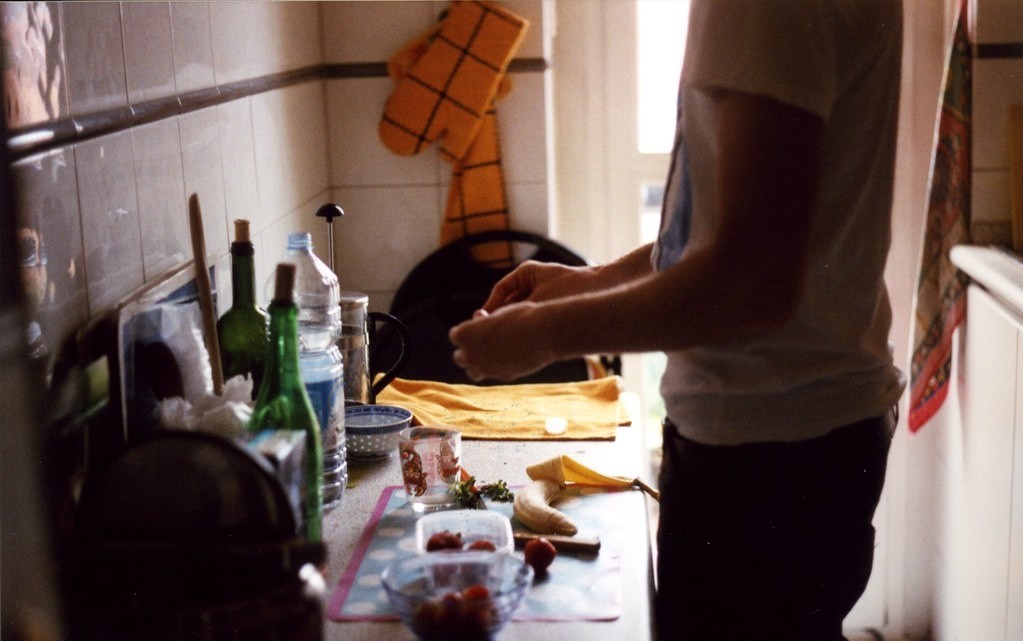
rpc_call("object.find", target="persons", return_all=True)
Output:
[449,0,909,641]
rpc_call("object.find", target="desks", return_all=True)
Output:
[314,388,658,640]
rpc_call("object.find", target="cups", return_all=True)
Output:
[398,425,464,515]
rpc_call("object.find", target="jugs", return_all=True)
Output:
[338,289,412,407]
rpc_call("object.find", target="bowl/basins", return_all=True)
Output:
[380,547,535,641]
[344,405,412,462]
[414,509,515,559]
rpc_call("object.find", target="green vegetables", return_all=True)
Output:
[449,475,514,510]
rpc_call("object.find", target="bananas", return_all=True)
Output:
[512,455,660,537]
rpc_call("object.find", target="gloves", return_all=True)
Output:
[377,0,530,163]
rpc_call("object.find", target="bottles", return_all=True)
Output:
[246,262,325,554]
[215,217,272,403]
[263,232,348,511]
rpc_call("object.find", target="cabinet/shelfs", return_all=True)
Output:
[927,279,1023,641]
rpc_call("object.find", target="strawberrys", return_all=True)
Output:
[522,538,557,570]
[427,530,498,583]
[408,585,501,641]
